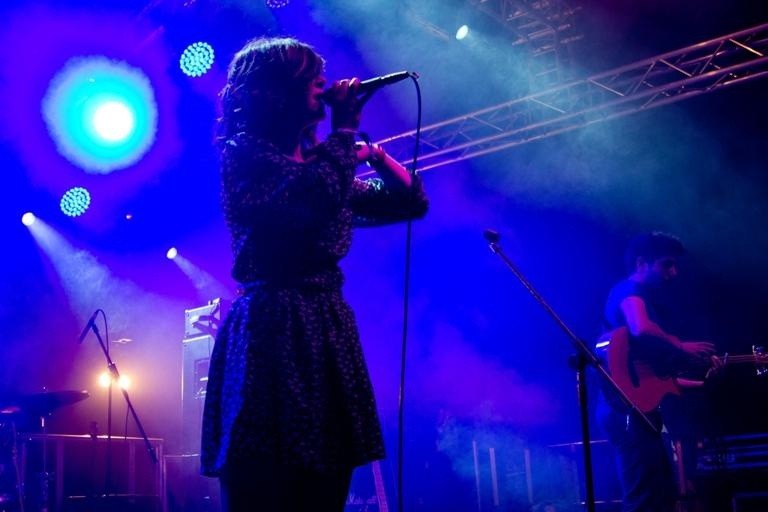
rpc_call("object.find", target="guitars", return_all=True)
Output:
[596,328,768,412]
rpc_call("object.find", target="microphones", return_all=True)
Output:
[324,71,409,107]
[79,312,97,344]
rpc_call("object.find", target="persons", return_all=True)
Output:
[198,40,431,512]
[594,229,725,512]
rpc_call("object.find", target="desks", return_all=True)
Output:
[18,431,164,511]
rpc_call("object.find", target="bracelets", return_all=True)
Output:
[366,145,385,167]
